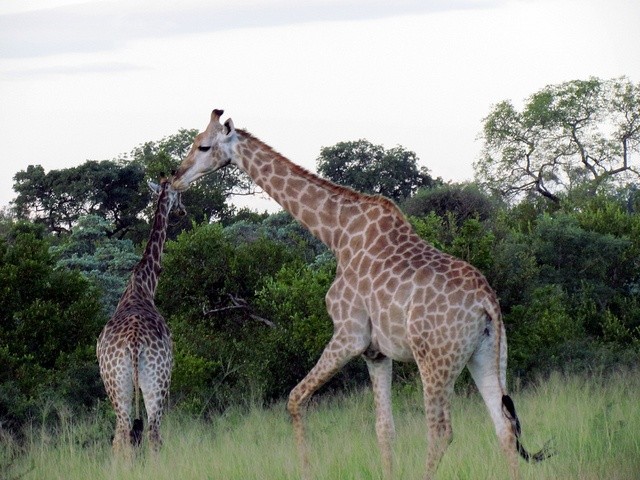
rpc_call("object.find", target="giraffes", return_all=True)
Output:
[96,169,187,468]
[168,108,558,480]
